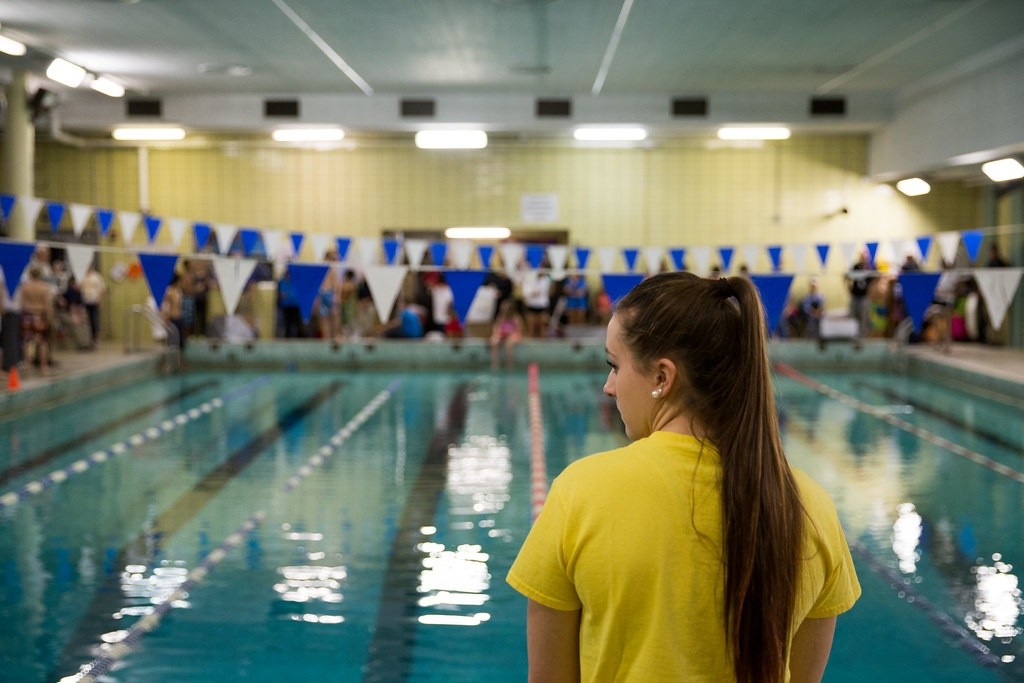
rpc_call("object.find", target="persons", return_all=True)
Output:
[788,282,823,338]
[147,251,454,347]
[488,258,588,344]
[506,272,864,683]
[0,247,112,377]
[847,252,875,334]
[985,242,1009,267]
[901,256,921,272]
[710,266,748,280]
